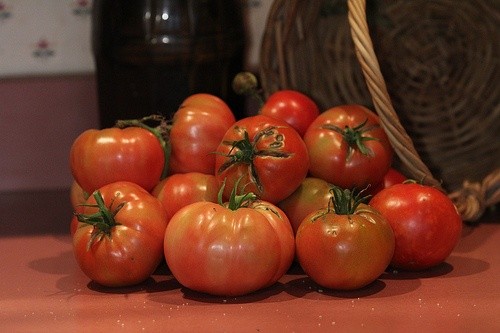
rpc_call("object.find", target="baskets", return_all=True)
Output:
[259,0,500,224]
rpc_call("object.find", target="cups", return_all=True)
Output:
[142,0,236,91]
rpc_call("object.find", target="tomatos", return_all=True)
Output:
[68,89,463,295]
